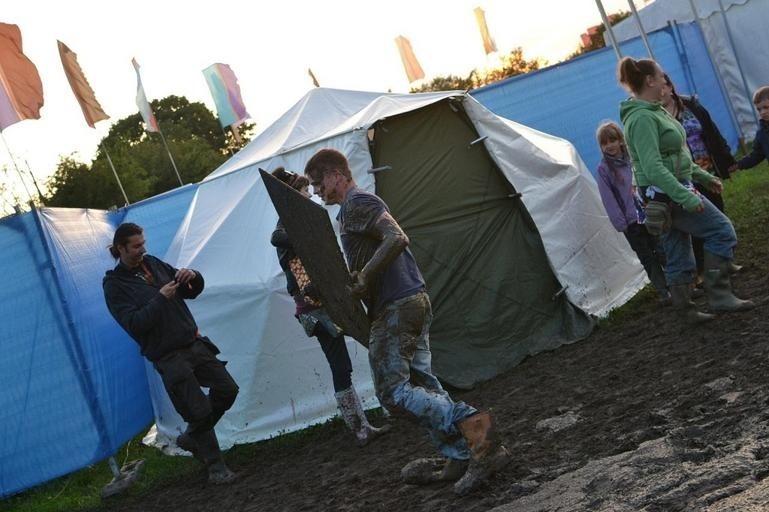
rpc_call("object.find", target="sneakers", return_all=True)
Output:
[208,466,240,485]
[176,432,206,464]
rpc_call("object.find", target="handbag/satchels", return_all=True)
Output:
[644,201,672,236]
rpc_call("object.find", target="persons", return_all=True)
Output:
[617,53,754,327]
[594,119,678,304]
[302,148,515,495]
[102,221,242,486]
[272,168,393,449]
[659,72,744,290]
[728,85,769,172]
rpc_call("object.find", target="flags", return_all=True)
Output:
[308,68,321,88]
[1,22,45,129]
[394,35,426,83]
[56,39,112,128]
[201,62,251,132]
[131,57,161,132]
[472,7,497,54]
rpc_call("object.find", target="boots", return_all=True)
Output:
[399,456,471,485]
[451,406,516,494]
[669,282,719,329]
[333,385,391,447]
[703,249,755,313]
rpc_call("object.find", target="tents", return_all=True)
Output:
[140,84,653,462]
